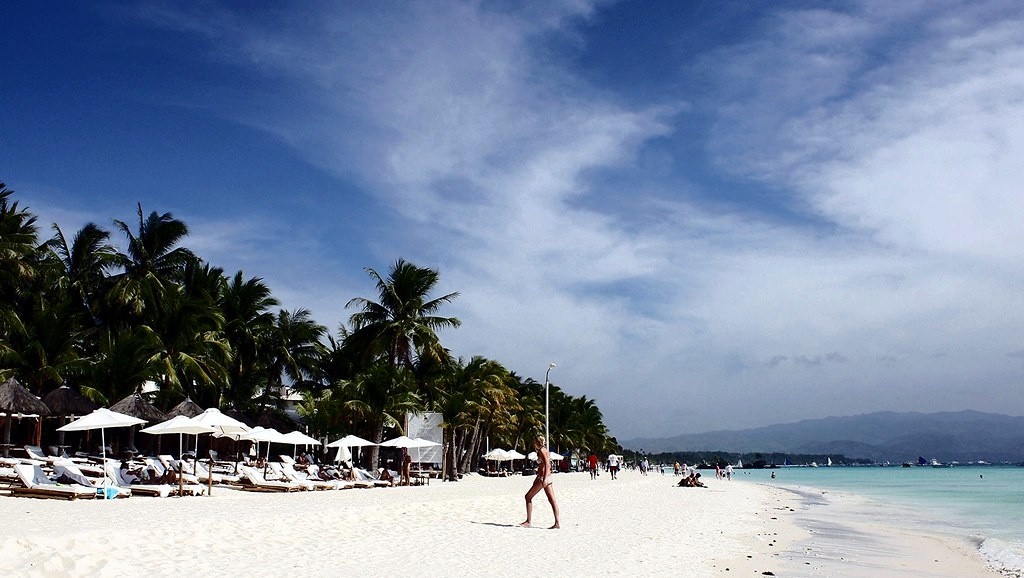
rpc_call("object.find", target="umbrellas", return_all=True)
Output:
[138,407,442,499]
[481,448,563,470]
[55,408,149,499]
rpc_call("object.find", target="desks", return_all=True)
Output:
[415,477,430,486]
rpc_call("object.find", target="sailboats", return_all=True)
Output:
[826,456,832,466]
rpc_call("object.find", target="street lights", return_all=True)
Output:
[546,364,557,451]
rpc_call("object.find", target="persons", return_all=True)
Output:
[521,435,560,528]
[485,461,491,476]
[586,449,775,487]
[120,444,411,485]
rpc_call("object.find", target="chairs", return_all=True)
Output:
[0,444,416,500]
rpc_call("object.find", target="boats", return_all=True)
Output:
[978,460,992,465]
[901,456,960,468]
[803,460,819,468]
